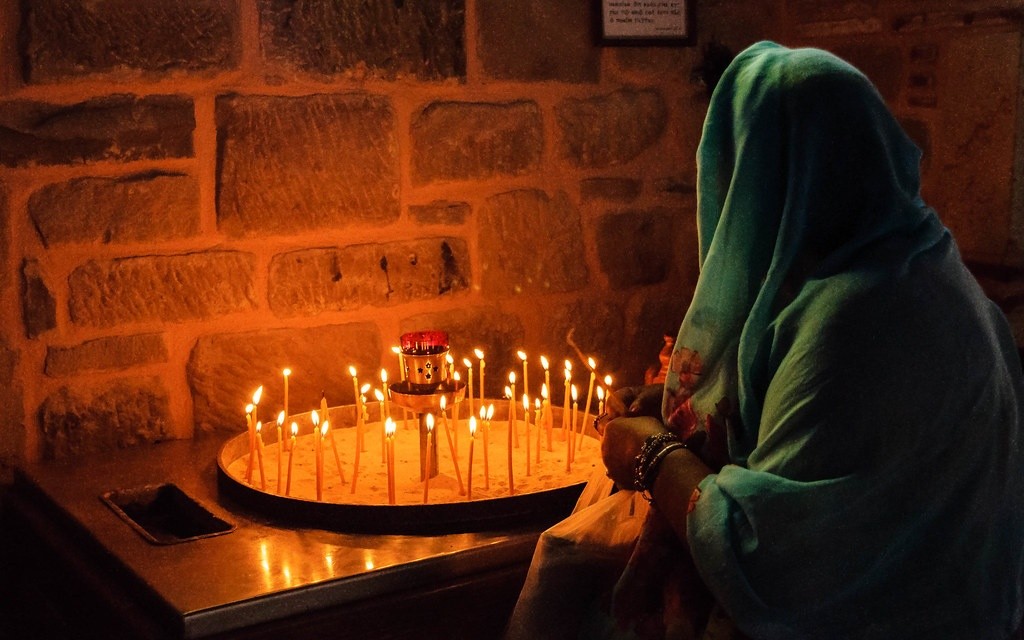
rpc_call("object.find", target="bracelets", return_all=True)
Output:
[634,432,688,502]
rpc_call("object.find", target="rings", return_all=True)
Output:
[593,413,614,437]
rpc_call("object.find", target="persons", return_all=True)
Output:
[549,39,1022,639]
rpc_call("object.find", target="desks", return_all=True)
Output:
[0,397,601,640]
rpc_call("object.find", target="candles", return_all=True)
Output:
[322,390,346,484]
[392,345,409,430]
[255,421,267,491]
[276,410,285,493]
[248,384,263,484]
[374,388,387,463]
[282,368,291,449]
[384,417,393,504]
[320,390,326,429]
[391,422,396,504]
[404,358,418,430]
[423,347,613,503]
[320,420,329,490]
[381,368,391,417]
[245,403,254,480]
[348,365,371,495]
[311,409,322,502]
[286,422,299,495]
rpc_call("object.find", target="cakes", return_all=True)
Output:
[226,418,617,503]
